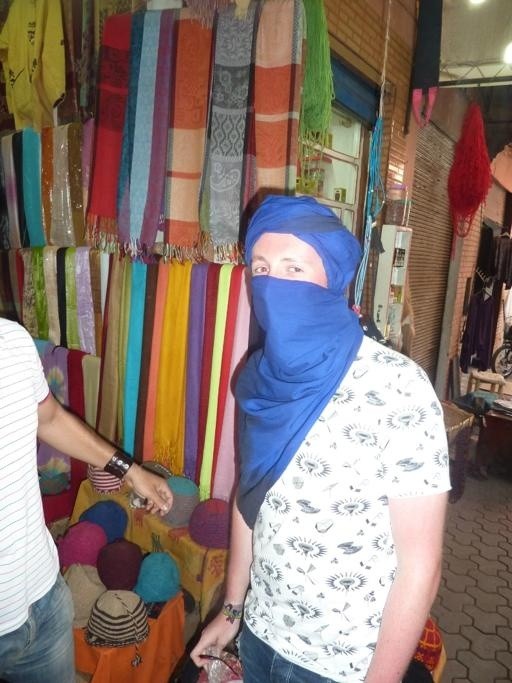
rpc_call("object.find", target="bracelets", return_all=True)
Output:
[220,603,247,623]
[103,451,133,479]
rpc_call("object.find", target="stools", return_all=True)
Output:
[464,368,504,397]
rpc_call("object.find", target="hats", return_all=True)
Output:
[53,456,236,653]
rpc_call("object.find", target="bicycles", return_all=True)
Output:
[491,327,512,379]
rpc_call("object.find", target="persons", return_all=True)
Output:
[0,314,172,681]
[189,191,455,682]
